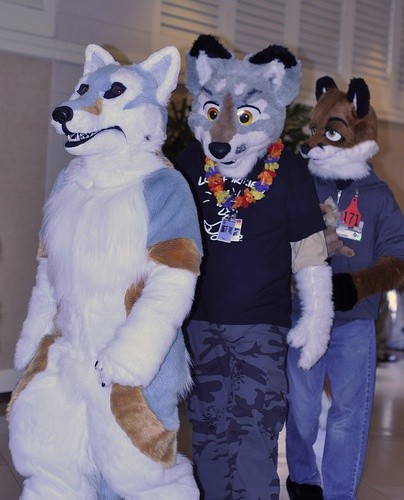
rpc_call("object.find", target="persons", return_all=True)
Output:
[284,76,404,499]
[174,34,335,499]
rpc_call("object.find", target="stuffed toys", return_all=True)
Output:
[6,44,203,500]
